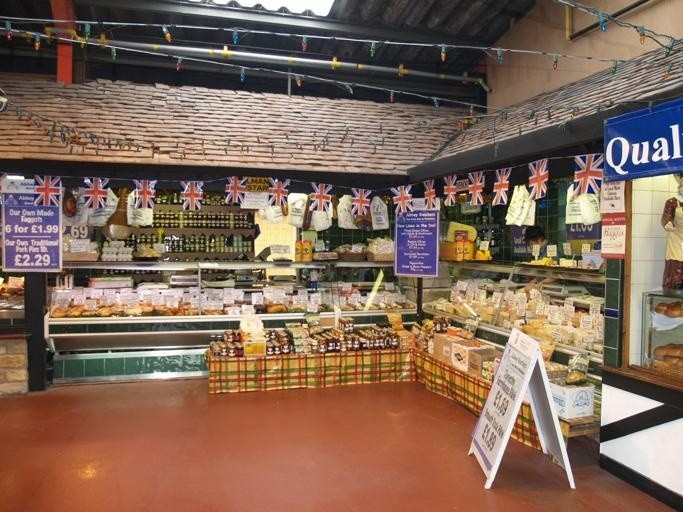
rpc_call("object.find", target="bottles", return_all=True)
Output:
[202,191,225,206]
[435,316,452,333]
[209,331,243,358]
[156,190,182,204]
[125,233,156,249]
[308,317,399,354]
[164,233,215,253]
[265,329,293,355]
[152,210,253,229]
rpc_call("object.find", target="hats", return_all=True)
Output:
[523,225,543,241]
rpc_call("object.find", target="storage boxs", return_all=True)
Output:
[452,340,495,373]
[549,379,595,419]
[468,350,500,377]
[432,333,464,366]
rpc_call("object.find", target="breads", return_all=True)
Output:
[654,343,683,368]
[51,308,66,317]
[81,309,96,316]
[112,305,124,314]
[124,305,143,316]
[66,305,81,316]
[203,309,222,316]
[96,306,111,316]
[165,302,198,317]
[655,302,683,317]
[141,304,154,312]
[154,305,168,312]
[267,304,286,313]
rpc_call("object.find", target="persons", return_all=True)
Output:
[660,171,683,294]
[524,227,562,292]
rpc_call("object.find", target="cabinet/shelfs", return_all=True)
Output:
[44,262,419,381]
[641,287,683,376]
[420,259,604,376]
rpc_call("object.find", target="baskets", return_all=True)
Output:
[339,252,365,261]
[367,252,394,261]
[652,360,683,380]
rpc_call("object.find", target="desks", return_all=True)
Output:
[205,348,413,391]
[412,348,601,467]
